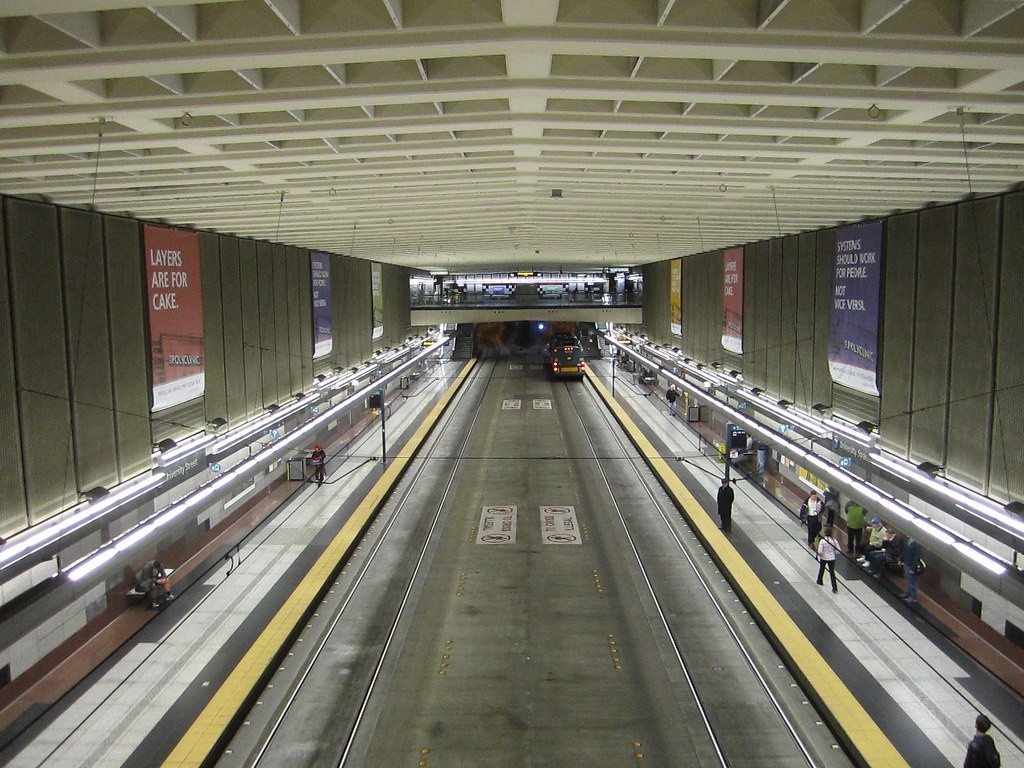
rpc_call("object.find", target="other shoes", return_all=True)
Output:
[719,527,724,529]
[904,597,917,603]
[863,561,871,567]
[809,541,814,549]
[724,528,731,532]
[900,593,909,597]
[824,523,832,527]
[672,413,676,416]
[846,549,853,553]
[856,555,866,562]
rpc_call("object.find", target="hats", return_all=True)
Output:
[871,516,880,525]
[722,478,729,483]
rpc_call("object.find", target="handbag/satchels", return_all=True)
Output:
[914,559,926,575]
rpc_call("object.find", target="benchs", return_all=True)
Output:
[751,460,849,544]
[895,573,1024,691]
[0,377,416,749]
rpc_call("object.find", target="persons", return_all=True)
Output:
[135,560,175,609]
[666,384,681,416]
[615,348,630,370]
[717,479,734,532]
[736,434,752,454]
[845,498,868,556]
[963,714,1001,768]
[756,439,767,474]
[817,527,841,593]
[312,444,327,487]
[799,490,825,549]
[638,366,647,383]
[822,485,840,527]
[856,516,904,578]
[897,534,922,603]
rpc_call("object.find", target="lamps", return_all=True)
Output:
[75,486,109,503]
[751,387,765,397]
[206,419,228,434]
[264,406,286,416]
[149,438,181,453]
[1003,500,1024,523]
[334,328,432,374]
[855,421,878,436]
[916,461,943,480]
[729,370,745,377]
[813,404,832,418]
[619,326,722,371]
[778,399,794,410]
[316,373,327,385]
[291,392,306,401]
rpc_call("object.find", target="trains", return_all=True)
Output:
[548,331,586,382]
